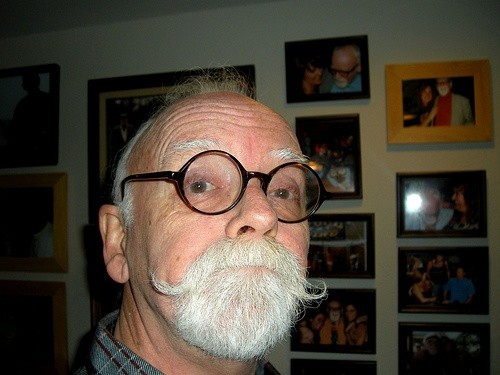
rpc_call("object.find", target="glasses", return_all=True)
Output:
[120,150,332,224]
[329,63,360,77]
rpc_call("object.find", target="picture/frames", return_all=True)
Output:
[0,62,61,168]
[396,170,487,238]
[385,59,493,144]
[0,280,69,375]
[290,289,377,355]
[397,245,490,315]
[398,322,491,375]
[284,35,370,104]
[303,213,376,279]
[0,172,69,273]
[295,113,363,201]
[88,64,256,226]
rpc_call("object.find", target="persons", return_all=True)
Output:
[402,73,476,127]
[410,333,476,374]
[295,298,369,347]
[287,40,363,92]
[76,73,326,375]
[403,252,480,309]
[401,180,482,231]
